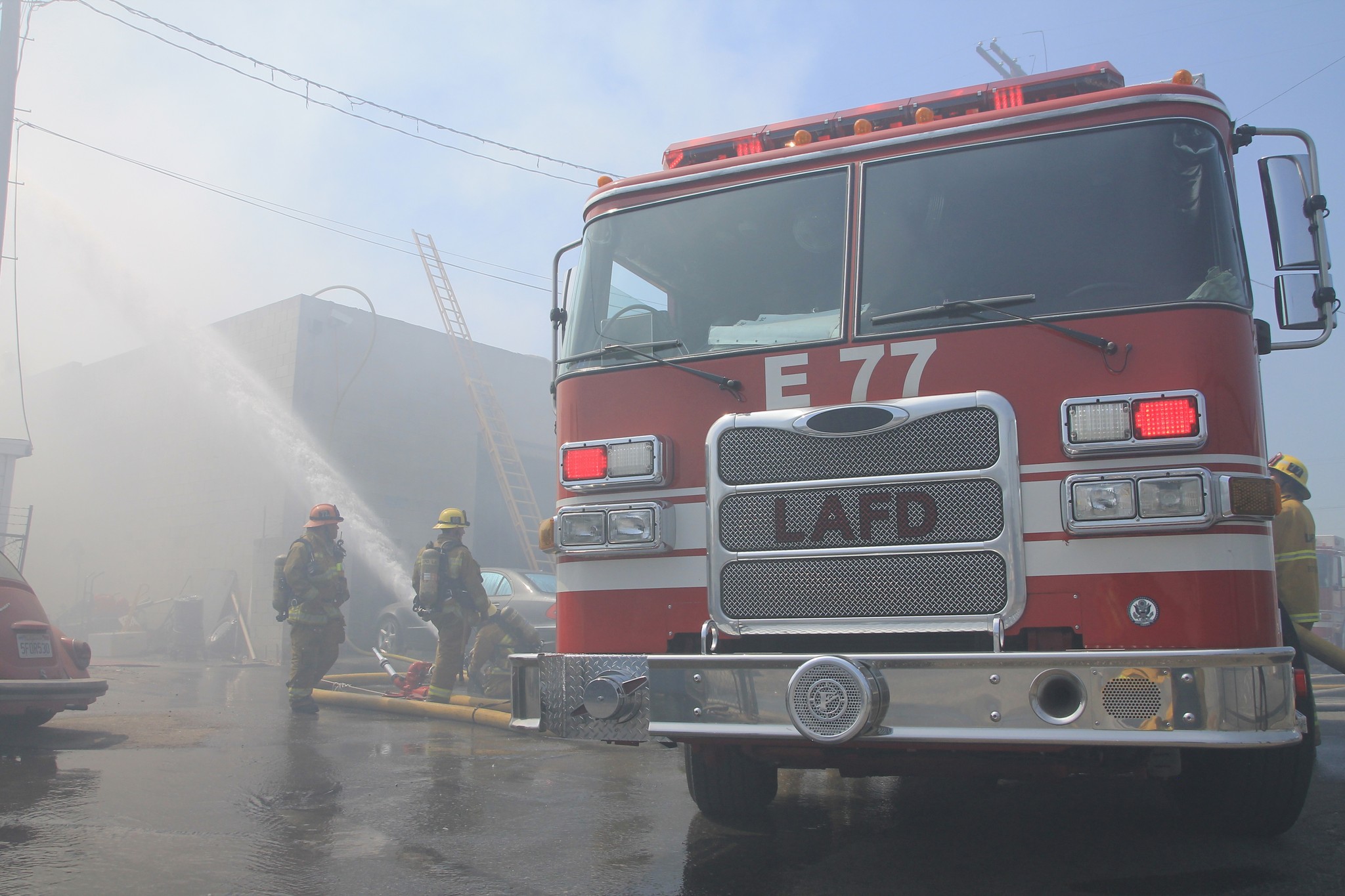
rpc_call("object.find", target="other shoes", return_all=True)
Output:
[285,680,290,688]
[292,703,320,713]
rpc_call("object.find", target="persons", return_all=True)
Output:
[1264,452,1319,631]
[412,509,489,704]
[466,600,532,688]
[285,505,352,714]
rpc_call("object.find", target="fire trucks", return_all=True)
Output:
[541,41,1340,834]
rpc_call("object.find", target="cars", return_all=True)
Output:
[378,568,556,656]
[0,548,107,733]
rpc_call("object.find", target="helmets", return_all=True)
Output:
[478,601,497,618]
[432,508,470,530]
[1267,452,1311,500]
[302,503,344,528]
